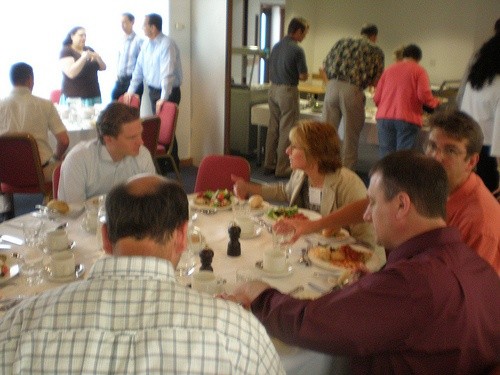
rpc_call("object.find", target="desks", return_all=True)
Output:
[250,98,432,170]
[47,105,108,163]
[0,192,379,375]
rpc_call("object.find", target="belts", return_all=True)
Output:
[41,160,51,169]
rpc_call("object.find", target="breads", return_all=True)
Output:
[315,246,345,261]
[249,194,263,208]
[46,200,69,215]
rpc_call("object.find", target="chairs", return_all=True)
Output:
[0,90,252,225]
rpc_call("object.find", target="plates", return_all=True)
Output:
[308,272,341,293]
[266,207,322,223]
[306,244,381,272]
[0,252,21,284]
[188,195,239,211]
[253,259,295,277]
[33,204,84,219]
[47,263,85,282]
[39,240,76,253]
[314,228,349,241]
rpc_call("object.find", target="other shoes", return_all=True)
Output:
[262,167,289,179]
[0,211,15,223]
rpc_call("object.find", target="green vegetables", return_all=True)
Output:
[268,205,298,221]
[203,189,233,208]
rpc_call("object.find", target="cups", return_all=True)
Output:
[232,199,264,239]
[19,249,46,287]
[56,103,104,122]
[271,225,295,258]
[263,248,286,272]
[236,266,263,283]
[45,231,69,251]
[192,271,216,292]
[49,252,75,277]
[21,220,41,247]
[175,213,205,277]
[83,196,104,229]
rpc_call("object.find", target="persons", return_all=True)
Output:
[262,16,310,182]
[106,12,144,113]
[274,109,500,279]
[56,101,158,219]
[374,41,448,161]
[454,18,500,204]
[124,13,183,175]
[58,26,106,106]
[231,119,377,255]
[0,172,291,375]
[319,22,385,172]
[239,149,500,375]
[0,61,70,184]
